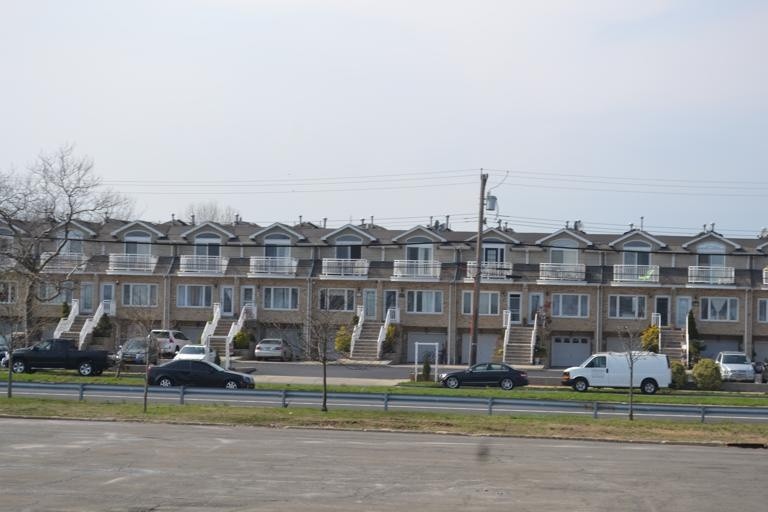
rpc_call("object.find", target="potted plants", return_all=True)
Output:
[534,351,540,365]
[382,335,401,364]
[233,331,250,360]
[93,312,114,351]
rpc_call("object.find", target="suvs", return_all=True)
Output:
[148,330,193,356]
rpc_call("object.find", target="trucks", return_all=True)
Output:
[561,350,673,394]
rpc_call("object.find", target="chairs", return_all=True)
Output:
[639,269,654,281]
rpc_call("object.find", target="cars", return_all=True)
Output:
[439,362,528,391]
[714,350,756,383]
[173,345,219,366]
[117,337,160,364]
[144,358,256,389]
[0,335,10,361]
[254,337,294,361]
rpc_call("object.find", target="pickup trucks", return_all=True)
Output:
[12,338,115,377]
